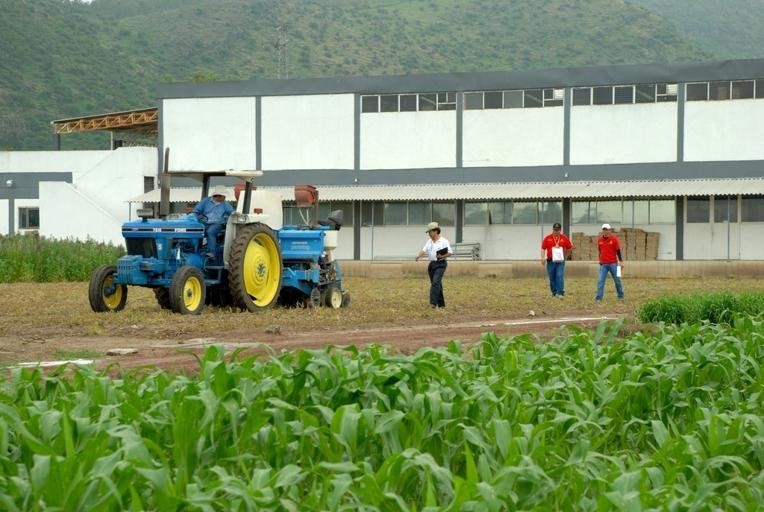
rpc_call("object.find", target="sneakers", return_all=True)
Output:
[206,253,215,262]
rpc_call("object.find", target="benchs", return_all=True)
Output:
[443,243,482,261]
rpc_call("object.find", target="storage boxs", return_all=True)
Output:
[570,227,661,261]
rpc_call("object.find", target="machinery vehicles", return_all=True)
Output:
[87,147,350,316]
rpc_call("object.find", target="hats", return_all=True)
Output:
[426,222,438,233]
[213,185,227,196]
[602,224,610,229]
[553,223,561,229]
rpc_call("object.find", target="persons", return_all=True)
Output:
[595,223,625,303]
[415,222,453,309]
[541,223,574,301]
[188,184,234,260]
